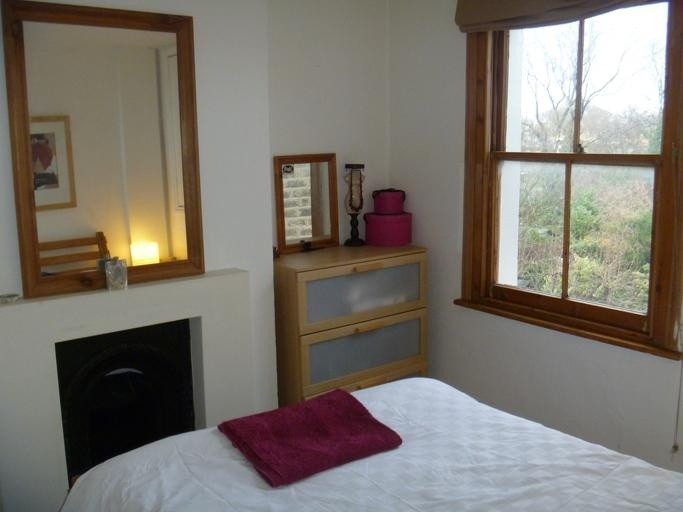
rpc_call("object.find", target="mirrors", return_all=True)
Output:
[0,0,211,301]
[271,152,341,254]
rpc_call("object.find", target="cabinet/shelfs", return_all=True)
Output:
[276,244,430,404]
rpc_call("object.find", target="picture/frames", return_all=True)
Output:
[28,115,80,213]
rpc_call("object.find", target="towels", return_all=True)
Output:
[216,382,408,487]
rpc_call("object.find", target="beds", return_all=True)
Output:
[63,373,683,512]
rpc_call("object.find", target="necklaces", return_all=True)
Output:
[349,170,363,213]
